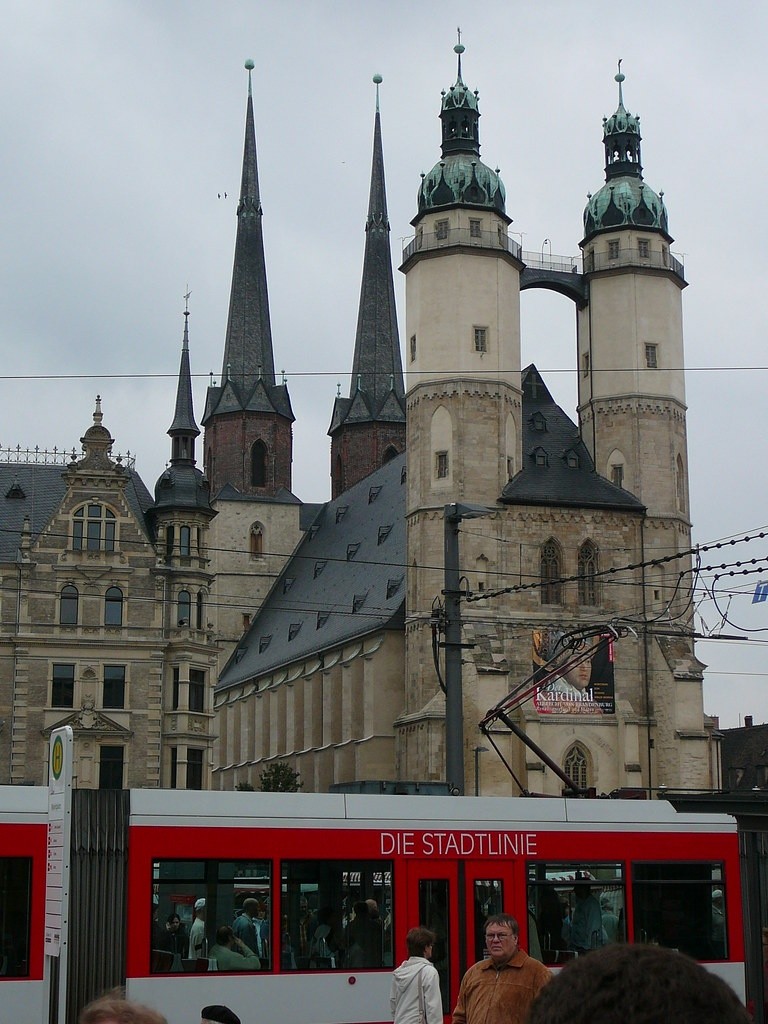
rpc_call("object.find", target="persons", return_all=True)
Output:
[453,912,556,1024]
[188,898,206,960]
[538,630,603,715]
[200,1004,241,1024]
[389,927,444,1024]
[158,913,189,966]
[250,887,393,969]
[207,926,261,970]
[152,902,160,949]
[527,944,755,1024]
[476,870,726,964]
[232,898,261,960]
[78,985,168,1024]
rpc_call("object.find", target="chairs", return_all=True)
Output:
[315,956,336,969]
[281,952,298,970]
[542,950,558,964]
[210,957,218,971]
[559,951,578,965]
[195,957,212,972]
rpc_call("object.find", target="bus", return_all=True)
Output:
[0,623,749,1024]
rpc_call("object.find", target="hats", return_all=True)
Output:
[712,890,722,902]
[195,898,205,910]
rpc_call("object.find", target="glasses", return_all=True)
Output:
[428,944,434,946]
[484,933,514,941]
